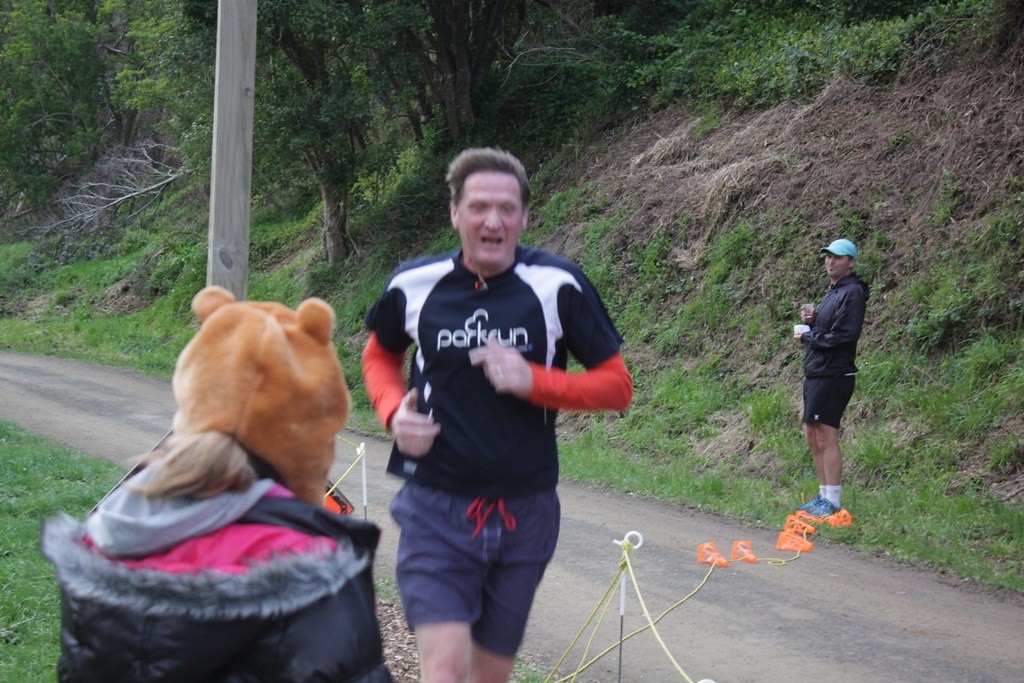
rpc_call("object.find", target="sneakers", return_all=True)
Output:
[810,498,843,516]
[797,496,822,510]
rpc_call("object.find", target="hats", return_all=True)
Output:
[171,285,351,509]
[820,239,857,258]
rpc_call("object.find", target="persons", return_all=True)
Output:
[360,146,631,683]
[793,238,867,525]
[42,286,397,682]
